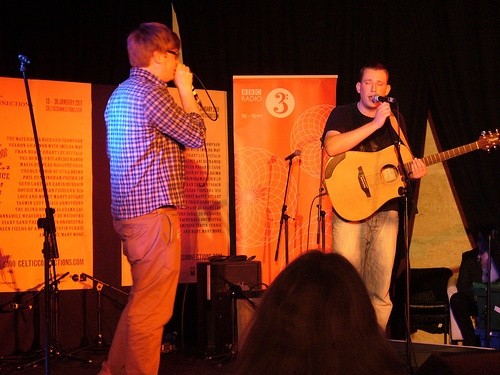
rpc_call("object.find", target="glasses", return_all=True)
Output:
[165,50,180,59]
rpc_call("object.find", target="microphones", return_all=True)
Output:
[1,302,34,312]
[192,84,202,107]
[18,55,32,66]
[371,94,397,103]
[239,294,259,311]
[398,186,411,196]
[285,150,301,161]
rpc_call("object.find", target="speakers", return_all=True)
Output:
[217,288,265,361]
[196,260,262,358]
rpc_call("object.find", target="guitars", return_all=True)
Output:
[324,128,500,222]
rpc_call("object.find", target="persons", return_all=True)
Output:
[322,63,426,338]
[99,22,206,375]
[450,225,500,347]
[227,250,399,375]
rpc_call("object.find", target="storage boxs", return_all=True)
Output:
[471,283,500,347]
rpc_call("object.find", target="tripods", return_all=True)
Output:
[15,72,130,369]
